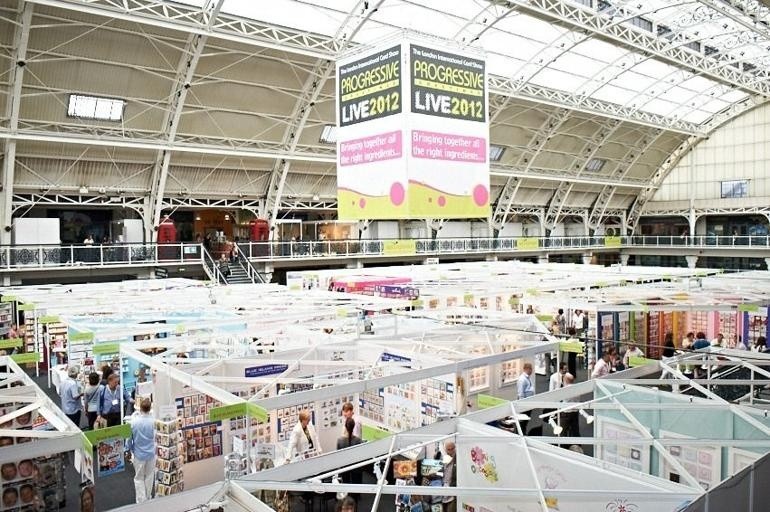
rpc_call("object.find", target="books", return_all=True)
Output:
[155,394,221,498]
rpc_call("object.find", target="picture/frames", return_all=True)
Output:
[656,431,722,489]
[596,416,651,477]
[725,447,762,478]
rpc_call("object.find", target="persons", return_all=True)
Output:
[284,409,324,465]
[0,322,26,373]
[542,309,770,453]
[100,367,113,386]
[61,366,85,428]
[436,440,456,512]
[83,372,105,430]
[0,380,43,511]
[96,374,135,428]
[128,397,158,504]
[176,229,250,284]
[98,439,122,472]
[516,362,536,434]
[82,234,126,262]
[340,401,363,442]
[334,495,359,511]
[80,486,95,512]
[337,417,365,502]
[278,229,326,256]
